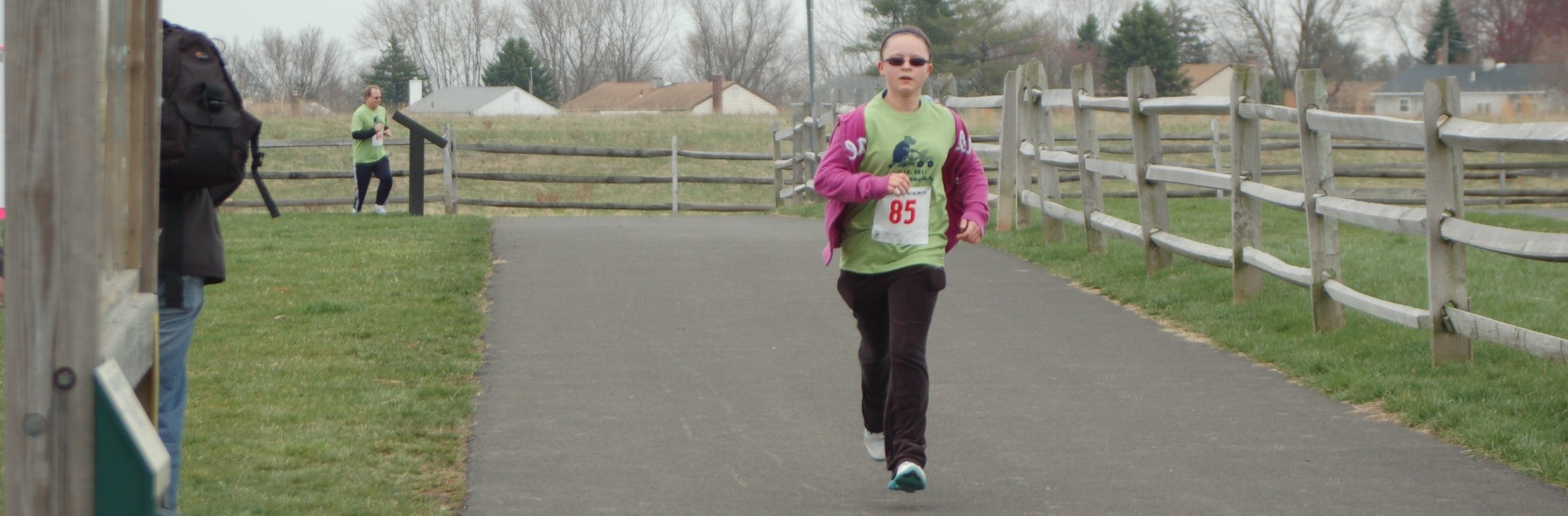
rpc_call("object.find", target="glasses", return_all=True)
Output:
[883,57,928,66]
[370,96,382,99]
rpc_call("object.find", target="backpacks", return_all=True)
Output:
[162,28,250,206]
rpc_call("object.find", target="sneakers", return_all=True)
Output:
[888,460,927,493]
[862,427,885,460]
[353,208,361,212]
[373,204,386,213]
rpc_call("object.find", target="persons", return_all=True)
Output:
[158,20,229,516]
[349,84,394,215]
[813,26,990,493]
